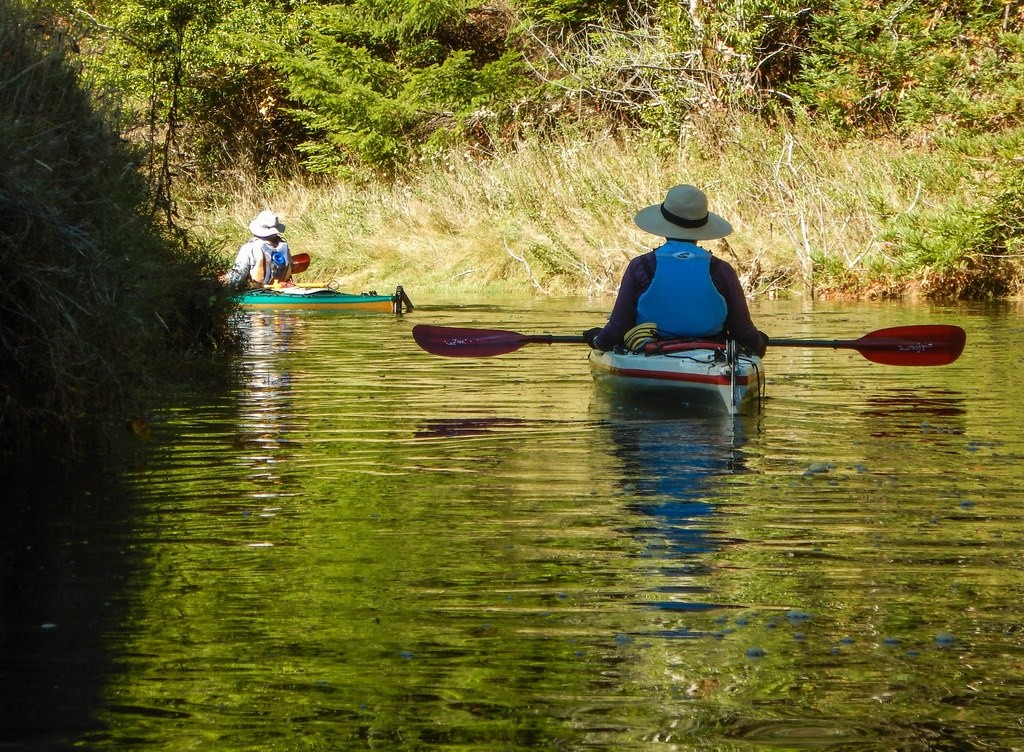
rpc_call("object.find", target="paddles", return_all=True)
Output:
[407,314,966,366]
[223,252,308,284]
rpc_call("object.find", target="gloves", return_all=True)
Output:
[583,327,603,349]
[759,331,769,343]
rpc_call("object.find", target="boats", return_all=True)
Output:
[586,346,766,420]
[205,282,416,315]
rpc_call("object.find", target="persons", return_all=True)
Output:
[580,183,769,357]
[217,209,293,289]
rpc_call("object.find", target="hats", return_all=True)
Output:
[248,211,285,237]
[633,185,733,240]
